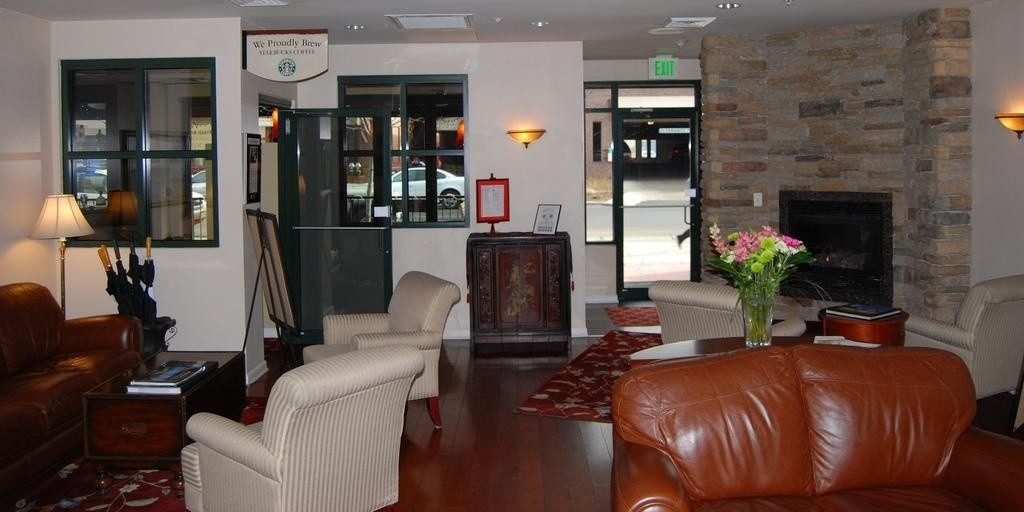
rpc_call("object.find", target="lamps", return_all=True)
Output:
[27,194,95,320]
[96,189,141,238]
[506,128,546,149]
[995,111,1023,140]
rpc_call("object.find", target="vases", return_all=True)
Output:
[738,281,774,347]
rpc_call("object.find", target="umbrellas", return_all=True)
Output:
[93,236,157,330]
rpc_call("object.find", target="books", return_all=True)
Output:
[126,359,219,396]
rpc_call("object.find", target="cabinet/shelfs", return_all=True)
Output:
[465,232,571,354]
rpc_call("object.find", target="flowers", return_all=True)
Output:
[706,222,817,344]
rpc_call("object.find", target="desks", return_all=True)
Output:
[820,300,909,348]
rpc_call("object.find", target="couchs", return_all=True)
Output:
[906,273,1024,401]
[649,280,806,346]
[0,282,143,512]
[607,344,1024,512]
[182,346,424,511]
[323,270,460,430]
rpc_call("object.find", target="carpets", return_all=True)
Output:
[514,331,661,424]
[604,307,660,329]
[0,395,269,511]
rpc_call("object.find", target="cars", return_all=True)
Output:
[72,168,208,225]
[392,168,465,208]
[191,162,211,201]
